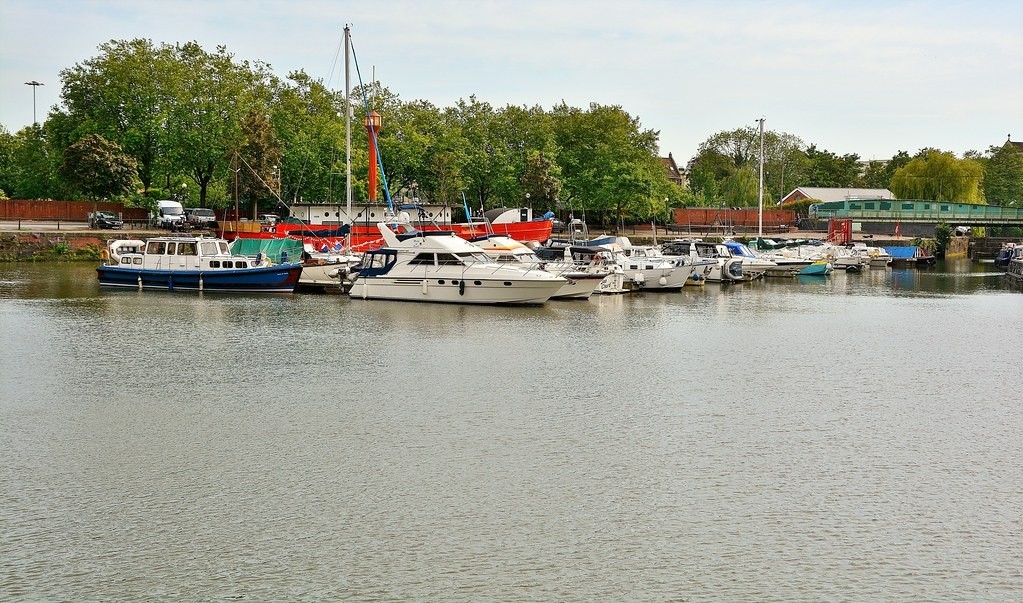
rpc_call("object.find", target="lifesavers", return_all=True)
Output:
[259,256,271,267]
[872,250,879,259]
[100,248,110,260]
[594,254,603,264]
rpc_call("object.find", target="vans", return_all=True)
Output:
[148,200,186,227]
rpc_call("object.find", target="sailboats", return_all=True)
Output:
[526,202,892,293]
[204,148,303,265]
[297,21,385,294]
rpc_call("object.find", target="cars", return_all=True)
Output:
[88,210,123,229]
[188,208,216,224]
[183,208,193,220]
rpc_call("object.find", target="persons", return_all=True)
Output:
[158,243,164,253]
[797,213,801,228]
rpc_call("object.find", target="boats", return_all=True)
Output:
[109,239,146,263]
[995,242,1023,282]
[329,207,583,304]
[95,235,303,294]
[459,193,610,298]
[216,66,555,254]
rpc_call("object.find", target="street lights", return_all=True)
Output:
[25,81,45,125]
[756,118,766,236]
